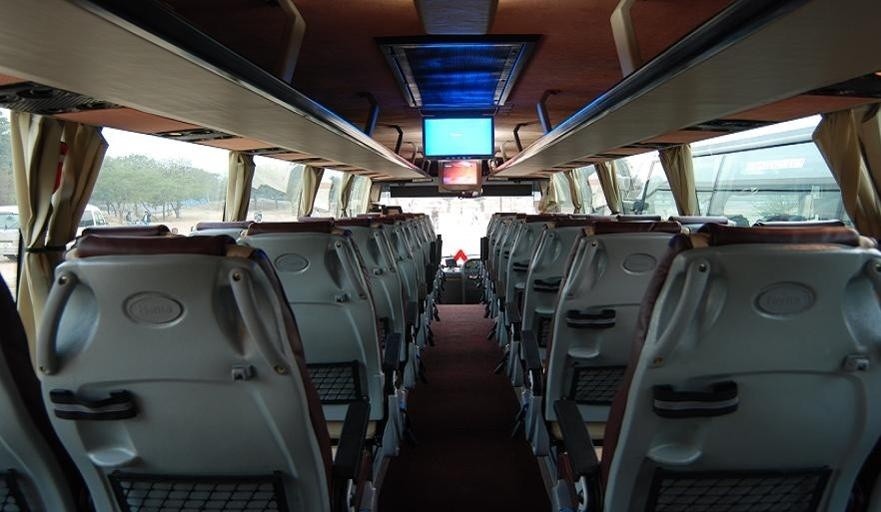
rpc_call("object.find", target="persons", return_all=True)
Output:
[126,210,132,225]
[140,210,152,225]
[254,209,264,224]
[172,228,179,234]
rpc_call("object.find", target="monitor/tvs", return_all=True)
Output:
[438,160,482,192]
[421,115,495,159]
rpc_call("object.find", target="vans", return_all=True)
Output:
[1,204,106,258]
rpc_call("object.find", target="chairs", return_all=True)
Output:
[480,214,880,511]
[0,212,442,512]
